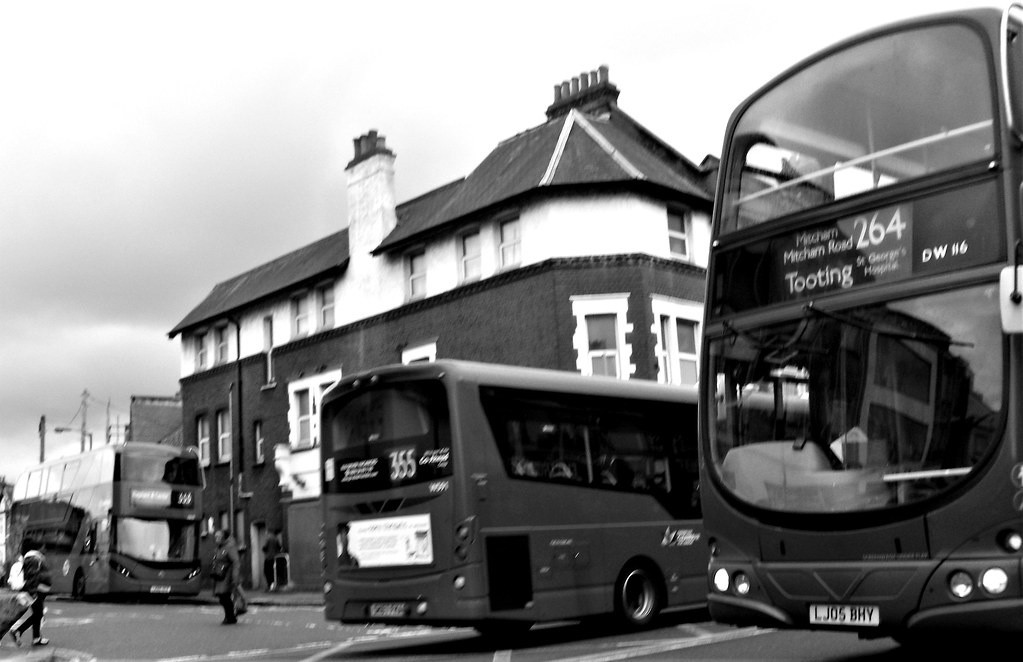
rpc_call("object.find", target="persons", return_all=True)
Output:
[10,538,52,646]
[827,397,869,464]
[262,529,281,593]
[212,530,247,625]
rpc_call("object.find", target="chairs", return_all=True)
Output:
[550,464,573,480]
[597,470,619,486]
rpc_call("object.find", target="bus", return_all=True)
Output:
[696,6,1023,662]
[319,359,809,642]
[10,442,205,602]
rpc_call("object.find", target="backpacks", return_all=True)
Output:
[7,550,45,590]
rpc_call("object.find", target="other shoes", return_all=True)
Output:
[33,637,49,645]
[10,630,22,646]
[223,617,238,625]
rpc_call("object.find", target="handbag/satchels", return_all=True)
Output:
[233,585,247,617]
[209,560,230,581]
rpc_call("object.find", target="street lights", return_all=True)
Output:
[55,427,92,450]
